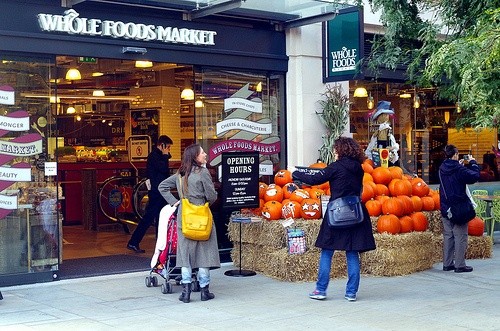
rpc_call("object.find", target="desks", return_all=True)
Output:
[472,194,500,237]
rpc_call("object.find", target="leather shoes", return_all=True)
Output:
[127,243,145,253]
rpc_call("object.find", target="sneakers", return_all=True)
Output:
[345,293,357,301]
[309,289,327,300]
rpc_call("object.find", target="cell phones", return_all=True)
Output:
[162,144,166,149]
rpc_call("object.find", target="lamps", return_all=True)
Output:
[182,89,194,100]
[413,95,420,108]
[50,62,105,113]
[353,82,375,109]
[135,61,153,67]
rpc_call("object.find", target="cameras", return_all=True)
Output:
[463,155,468,159]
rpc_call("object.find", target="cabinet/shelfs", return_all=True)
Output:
[368,109,394,141]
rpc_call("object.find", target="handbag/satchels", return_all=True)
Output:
[446,195,476,222]
[181,197,213,241]
[327,195,364,227]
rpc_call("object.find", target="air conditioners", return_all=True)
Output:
[455,101,462,113]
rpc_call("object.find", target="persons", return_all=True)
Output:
[291,136,376,302]
[127,135,173,253]
[158,144,220,303]
[438,145,480,272]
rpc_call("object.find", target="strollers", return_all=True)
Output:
[144,200,201,294]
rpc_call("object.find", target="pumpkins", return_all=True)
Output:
[241,160,332,221]
[361,159,484,237]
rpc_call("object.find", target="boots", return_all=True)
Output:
[178,283,192,303]
[201,284,215,301]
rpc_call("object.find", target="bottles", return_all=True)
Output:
[76,148,122,163]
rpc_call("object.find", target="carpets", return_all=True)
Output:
[59,253,152,277]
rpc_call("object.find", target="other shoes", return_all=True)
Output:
[443,264,455,271]
[454,265,473,272]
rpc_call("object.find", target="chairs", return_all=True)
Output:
[471,189,500,244]
